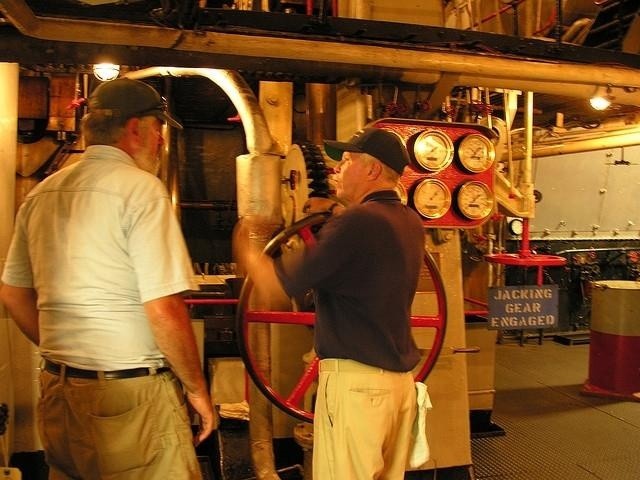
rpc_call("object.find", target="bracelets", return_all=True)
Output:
[327,202,344,215]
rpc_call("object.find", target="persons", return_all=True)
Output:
[275,130,423,480]
[1,77,221,480]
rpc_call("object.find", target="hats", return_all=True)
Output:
[322,126,411,175]
[88,76,185,131]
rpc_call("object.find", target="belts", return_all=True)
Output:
[45,359,170,381]
[319,357,389,374]
